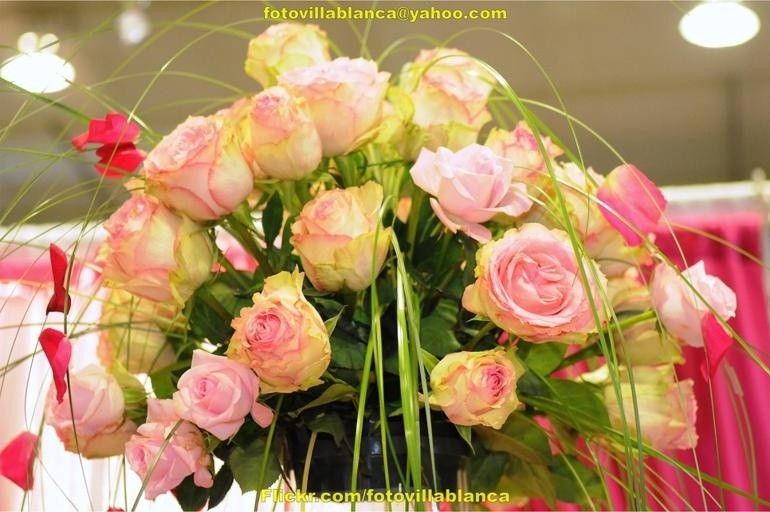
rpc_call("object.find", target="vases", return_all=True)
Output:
[285,417,430,498]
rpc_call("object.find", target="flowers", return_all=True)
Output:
[0,19,766,511]
